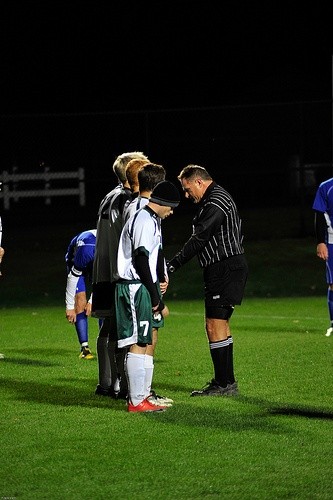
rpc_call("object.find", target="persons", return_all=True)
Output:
[165,164,244,397]
[0,183,4,359]
[93,152,179,414]
[65,229,97,360]
[312,178,333,336]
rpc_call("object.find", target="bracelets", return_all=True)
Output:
[166,262,174,272]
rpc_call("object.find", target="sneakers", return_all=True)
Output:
[190,379,238,396]
[147,390,173,407]
[80,345,94,359]
[127,397,166,414]
[93,383,112,396]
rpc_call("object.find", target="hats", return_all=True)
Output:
[150,180,182,208]
